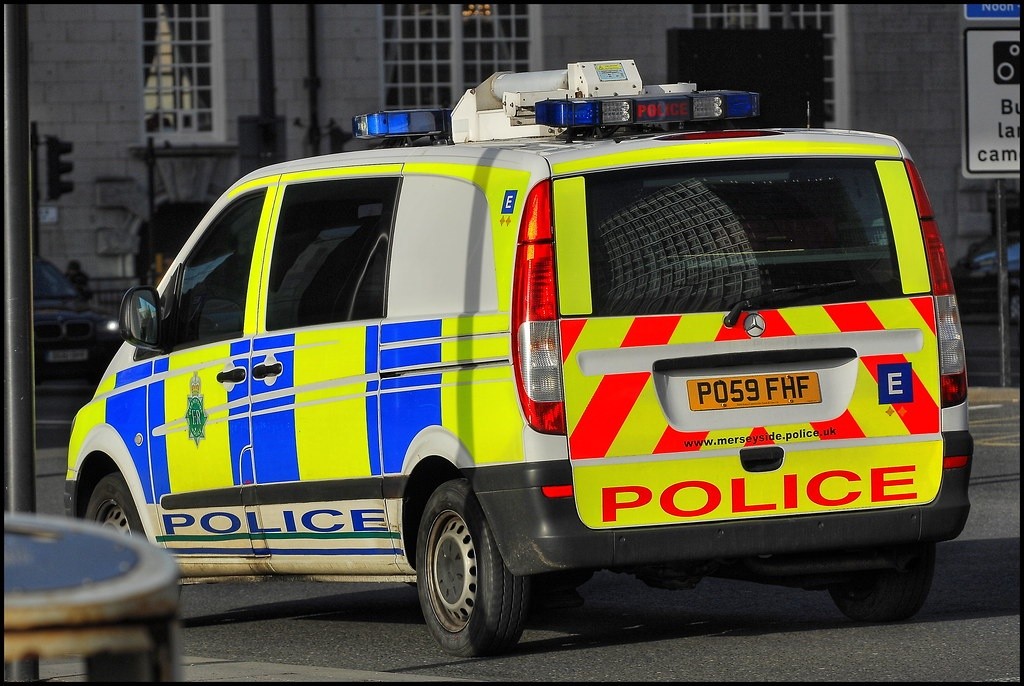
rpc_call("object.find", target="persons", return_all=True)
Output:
[65,259,94,299]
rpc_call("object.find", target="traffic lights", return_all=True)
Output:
[46,135,75,199]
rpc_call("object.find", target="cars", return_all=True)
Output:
[969,241,1021,324]
[33,258,125,383]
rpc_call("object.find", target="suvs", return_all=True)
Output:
[63,59,973,659]
